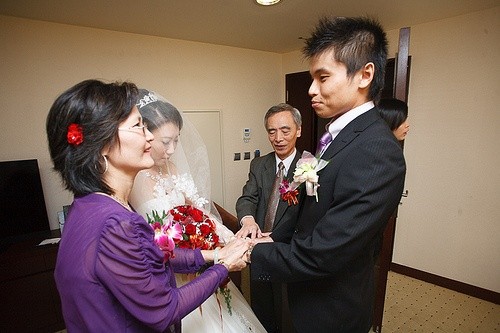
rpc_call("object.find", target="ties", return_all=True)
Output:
[313,130,332,159]
[262,162,285,233]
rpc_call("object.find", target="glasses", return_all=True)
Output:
[118,122,148,135]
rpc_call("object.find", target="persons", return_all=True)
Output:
[46,14,410,333]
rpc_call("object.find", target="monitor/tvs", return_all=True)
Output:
[0,159,50,254]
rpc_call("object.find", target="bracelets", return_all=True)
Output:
[214,247,229,269]
[246,245,255,261]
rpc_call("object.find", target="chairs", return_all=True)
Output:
[0,160,50,235]
[214,202,242,290]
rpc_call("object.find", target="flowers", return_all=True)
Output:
[279,180,299,206]
[166,206,219,251]
[66,123,83,145]
[146,210,183,259]
[293,151,329,202]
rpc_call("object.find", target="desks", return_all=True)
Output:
[1,228,65,333]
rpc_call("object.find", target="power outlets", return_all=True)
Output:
[234,152,240,161]
[244,151,250,161]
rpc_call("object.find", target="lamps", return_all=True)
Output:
[256,0,286,6]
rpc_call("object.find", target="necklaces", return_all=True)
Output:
[142,166,164,181]
[109,192,130,208]
